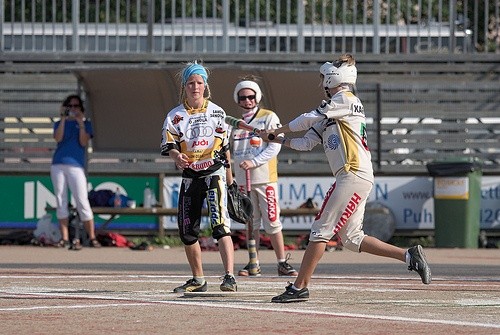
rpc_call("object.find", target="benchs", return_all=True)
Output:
[45,205,388,246]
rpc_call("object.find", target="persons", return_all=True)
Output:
[160,63,238,293]
[226,73,298,277]
[50,94,101,248]
[258,53,432,303]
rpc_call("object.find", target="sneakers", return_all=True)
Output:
[272,281,310,302]
[407,245,431,284]
[219,274,237,291]
[278,253,299,276]
[174,278,208,292]
[238,263,261,275]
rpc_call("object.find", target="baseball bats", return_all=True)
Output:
[245,168,258,275]
[224,116,277,140]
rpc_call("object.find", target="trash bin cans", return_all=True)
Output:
[427,158,483,248]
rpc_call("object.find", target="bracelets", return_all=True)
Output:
[283,139,286,144]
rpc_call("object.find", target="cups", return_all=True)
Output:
[129,201,136,209]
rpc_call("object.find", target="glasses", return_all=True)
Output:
[67,104,80,107]
[238,95,255,101]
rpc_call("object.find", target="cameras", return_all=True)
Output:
[64,110,76,117]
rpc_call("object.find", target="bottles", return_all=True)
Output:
[144,184,151,209]
[115,188,121,208]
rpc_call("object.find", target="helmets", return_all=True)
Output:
[319,62,357,88]
[233,81,262,103]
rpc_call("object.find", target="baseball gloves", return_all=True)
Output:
[226,180,254,224]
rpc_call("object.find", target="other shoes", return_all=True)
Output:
[90,239,101,247]
[54,239,71,247]
